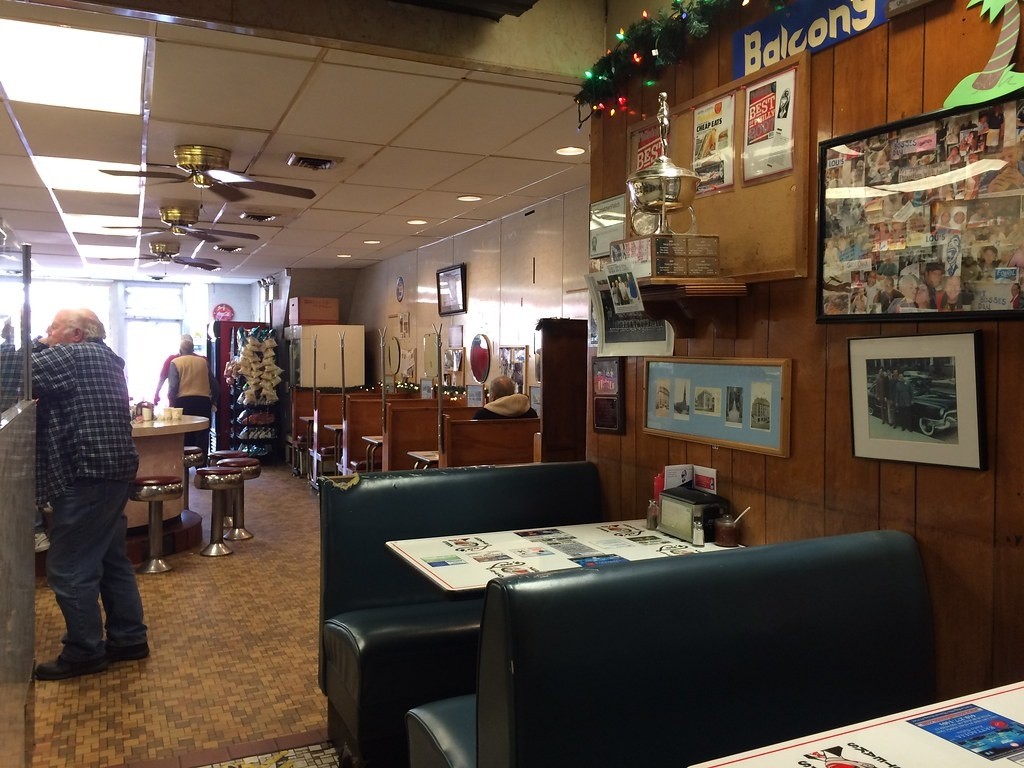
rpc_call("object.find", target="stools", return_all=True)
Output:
[128,476,184,572]
[182,446,262,557]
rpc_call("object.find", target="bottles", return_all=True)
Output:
[692,521,704,547]
[647,498,660,530]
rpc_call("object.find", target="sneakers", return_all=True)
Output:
[105,641,150,663]
[35,654,108,681]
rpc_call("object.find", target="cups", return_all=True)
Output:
[164,407,175,419]
[171,408,184,420]
[715,514,740,547]
[141,407,152,421]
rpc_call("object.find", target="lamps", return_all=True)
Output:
[257,275,279,302]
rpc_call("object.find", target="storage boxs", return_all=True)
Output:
[288,297,339,325]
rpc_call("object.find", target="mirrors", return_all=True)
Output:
[470,333,490,385]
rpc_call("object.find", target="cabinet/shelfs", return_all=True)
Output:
[283,323,365,445]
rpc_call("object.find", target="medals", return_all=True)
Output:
[609,91,721,280]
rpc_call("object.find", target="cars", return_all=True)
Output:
[867,375,957,437]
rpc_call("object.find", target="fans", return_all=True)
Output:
[100,241,222,281]
[98,145,317,203]
[103,204,261,244]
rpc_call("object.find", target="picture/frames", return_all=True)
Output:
[436,264,467,317]
[498,345,529,396]
[420,378,435,399]
[815,86,1024,326]
[846,329,987,471]
[442,347,466,388]
[466,384,486,407]
[591,355,626,437]
[642,356,792,459]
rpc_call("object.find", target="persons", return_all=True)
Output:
[473,376,539,419]
[1,307,151,681]
[821,96,1024,315]
[168,340,221,477]
[876,366,930,432]
[470,337,489,383]
[154,334,208,407]
[609,272,641,307]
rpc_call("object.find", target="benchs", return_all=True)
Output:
[319,458,937,768]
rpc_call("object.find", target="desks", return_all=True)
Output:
[299,417,439,480]
[386,517,749,597]
